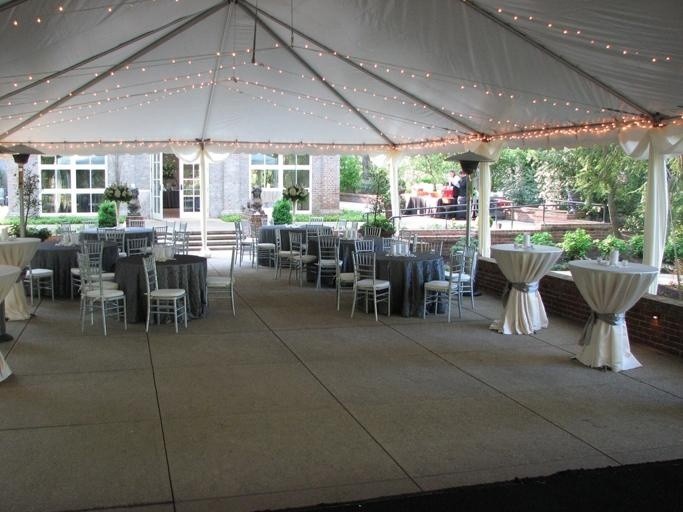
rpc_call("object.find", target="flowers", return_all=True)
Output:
[282,183,309,202]
[103,181,133,203]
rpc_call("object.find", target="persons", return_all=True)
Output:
[446,168,461,187]
[453,170,467,219]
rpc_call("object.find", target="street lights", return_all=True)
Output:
[1,144,43,237]
[445,151,494,273]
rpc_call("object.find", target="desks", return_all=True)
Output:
[567,259,659,372]
[489,243,563,335]
[0,236,42,383]
[163,189,179,208]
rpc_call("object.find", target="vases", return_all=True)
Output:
[292,200,298,218]
[115,202,122,227]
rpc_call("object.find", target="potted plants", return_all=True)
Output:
[126,188,145,227]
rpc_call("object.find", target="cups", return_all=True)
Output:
[0,227,8,242]
[151,242,174,260]
[609,247,619,266]
[522,232,530,247]
[59,231,80,246]
[337,218,358,241]
[11,235,15,241]
[389,238,410,256]
